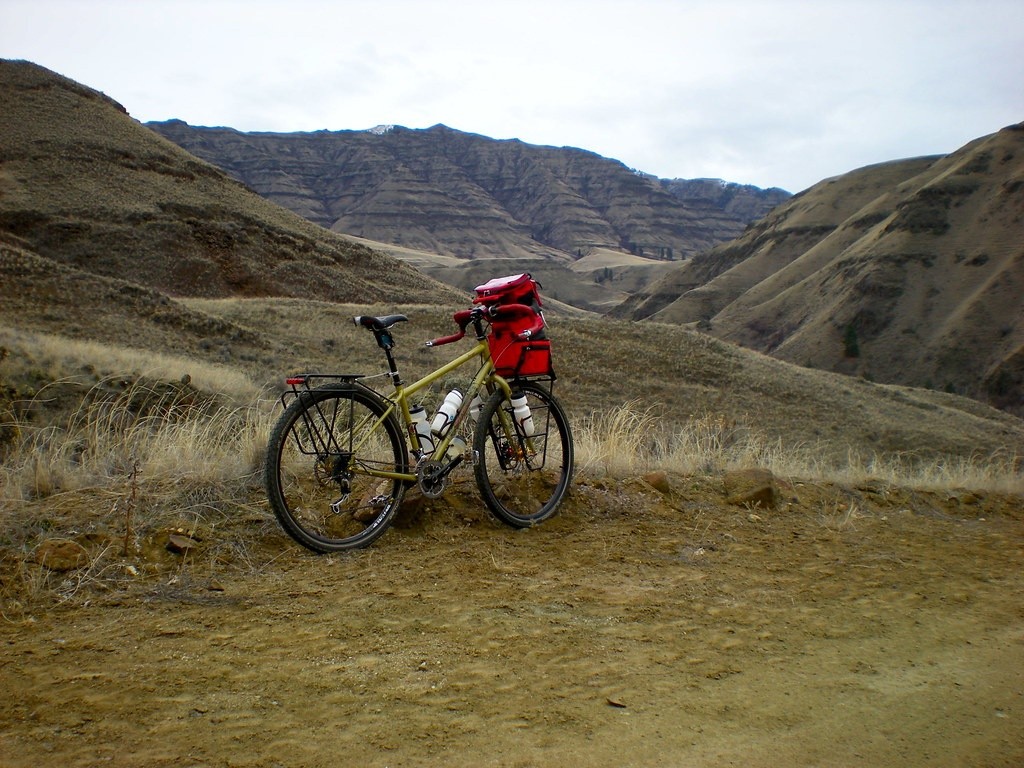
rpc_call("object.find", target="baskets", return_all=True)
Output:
[487,331,550,375]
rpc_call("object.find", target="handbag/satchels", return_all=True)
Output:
[473,272,547,340]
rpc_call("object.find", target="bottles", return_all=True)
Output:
[469,393,484,422]
[441,435,467,465]
[410,403,435,453]
[431,388,464,436]
[510,391,535,436]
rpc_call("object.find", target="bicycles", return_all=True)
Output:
[264,303,575,554]
